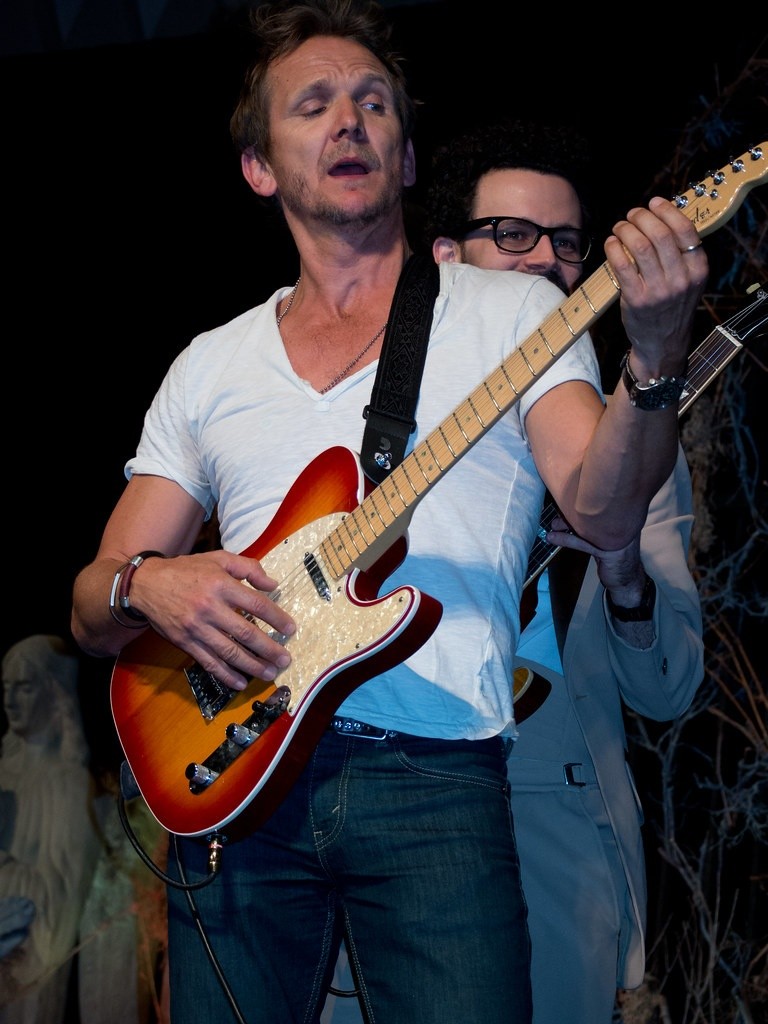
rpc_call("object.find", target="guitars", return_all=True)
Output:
[107,141,768,844]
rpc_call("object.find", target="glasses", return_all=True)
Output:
[452,216,599,265]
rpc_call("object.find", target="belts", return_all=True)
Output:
[332,717,503,755]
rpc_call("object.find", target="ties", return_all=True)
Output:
[524,497,563,577]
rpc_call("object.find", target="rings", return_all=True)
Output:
[680,241,704,254]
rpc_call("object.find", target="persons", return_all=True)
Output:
[72,0,706,1023]
[0,632,173,1024]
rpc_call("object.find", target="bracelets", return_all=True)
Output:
[119,550,167,621]
[109,563,150,629]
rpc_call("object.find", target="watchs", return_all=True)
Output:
[606,573,656,622]
[619,350,687,411]
